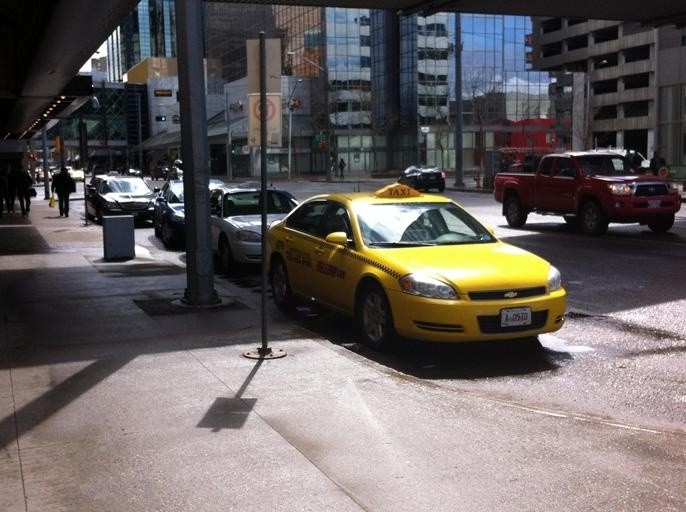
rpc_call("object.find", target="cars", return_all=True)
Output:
[398,165,446,193]
[34,158,186,225]
[155,178,227,248]
[205,183,300,276]
[268,179,567,351]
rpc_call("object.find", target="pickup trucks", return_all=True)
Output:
[491,148,682,236]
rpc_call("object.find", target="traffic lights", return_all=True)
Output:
[155,116,166,121]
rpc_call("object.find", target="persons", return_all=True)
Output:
[51,167,70,217]
[650,152,665,176]
[332,158,346,180]
[0,164,33,217]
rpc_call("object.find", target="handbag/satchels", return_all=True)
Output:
[48,196,56,208]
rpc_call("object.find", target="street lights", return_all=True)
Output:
[286,79,303,179]
[585,59,609,151]
